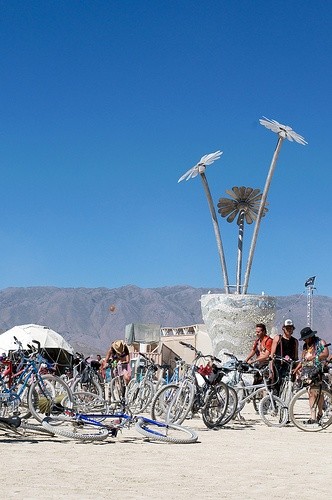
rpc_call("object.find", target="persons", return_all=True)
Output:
[96,355,103,363]
[291,327,332,423]
[244,324,274,385]
[270,319,299,416]
[102,340,131,384]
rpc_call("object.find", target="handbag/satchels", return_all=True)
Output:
[301,365,318,378]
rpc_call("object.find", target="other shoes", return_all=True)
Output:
[303,412,323,424]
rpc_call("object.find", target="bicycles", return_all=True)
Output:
[166,341,230,430]
[0,336,238,437]
[41,386,198,444]
[288,343,332,432]
[221,365,288,427]
[223,351,270,420]
[0,340,74,426]
[269,354,302,425]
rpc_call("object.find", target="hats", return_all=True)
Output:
[299,327,317,341]
[282,319,296,330]
[112,340,124,354]
